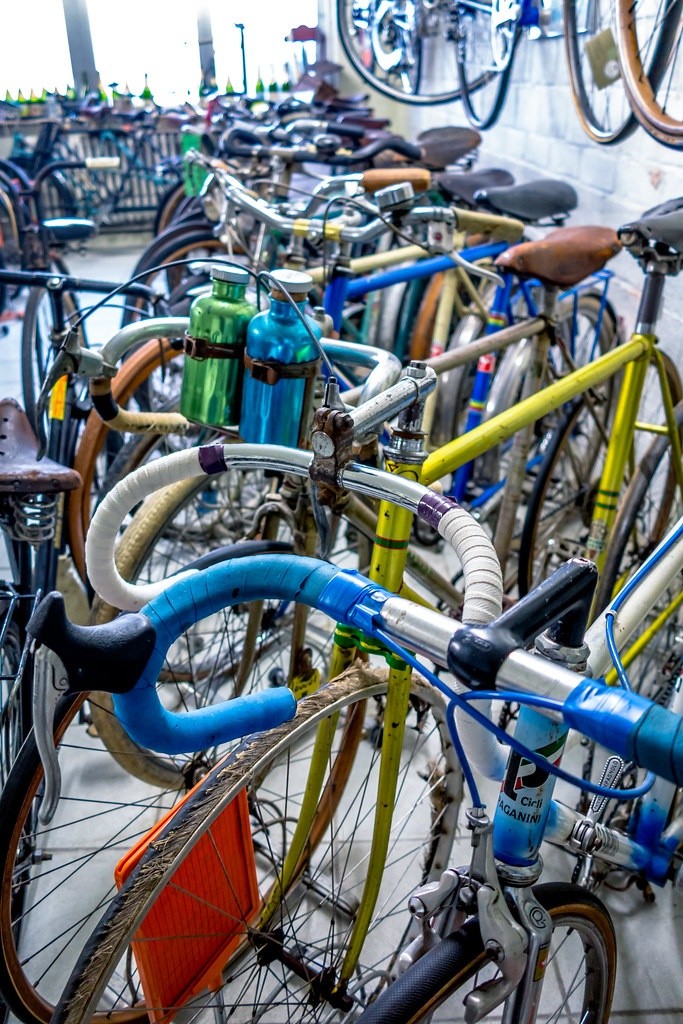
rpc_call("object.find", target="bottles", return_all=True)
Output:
[178,264,261,426]
[4,60,299,122]
[239,269,323,448]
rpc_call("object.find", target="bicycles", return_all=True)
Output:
[0,0,683,1024]
